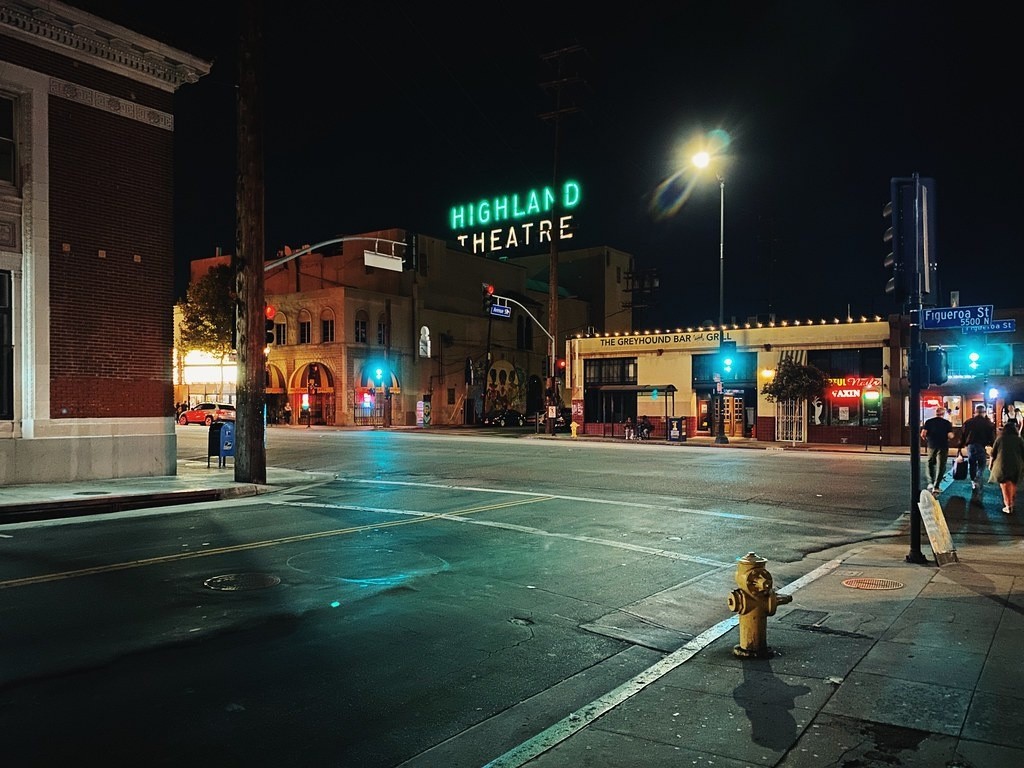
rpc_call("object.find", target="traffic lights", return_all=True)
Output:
[987,384,1005,400]
[482,282,493,313]
[556,359,565,380]
[402,234,417,269]
[883,176,940,299]
[967,332,986,376]
[309,363,317,379]
[721,340,737,377]
[542,355,550,377]
[374,367,383,381]
[264,302,275,343]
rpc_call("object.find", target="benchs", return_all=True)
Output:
[624,424,655,438]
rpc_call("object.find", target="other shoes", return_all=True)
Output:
[927,484,942,493]
[1002,506,1014,513]
[971,481,982,489]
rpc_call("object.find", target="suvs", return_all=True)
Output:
[178,403,238,426]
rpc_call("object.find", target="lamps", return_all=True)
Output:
[883,201,896,293]
[657,349,664,356]
[764,342,773,352]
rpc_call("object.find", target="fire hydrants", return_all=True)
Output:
[570,422,580,439]
[726,552,793,658]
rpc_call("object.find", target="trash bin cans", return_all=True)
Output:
[208,421,235,469]
[668,416,686,442]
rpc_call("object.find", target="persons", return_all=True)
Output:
[624,415,652,440]
[920,407,955,492]
[988,422,1024,514]
[176,400,188,423]
[283,402,292,426]
[958,405,995,489]
[1004,403,1023,437]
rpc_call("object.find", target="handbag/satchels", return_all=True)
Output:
[952,448,968,480]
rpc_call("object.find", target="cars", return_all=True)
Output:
[526,410,565,426]
[480,409,526,427]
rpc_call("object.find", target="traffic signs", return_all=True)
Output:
[922,304,1017,334]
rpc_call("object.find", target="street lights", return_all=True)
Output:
[691,151,733,442]
